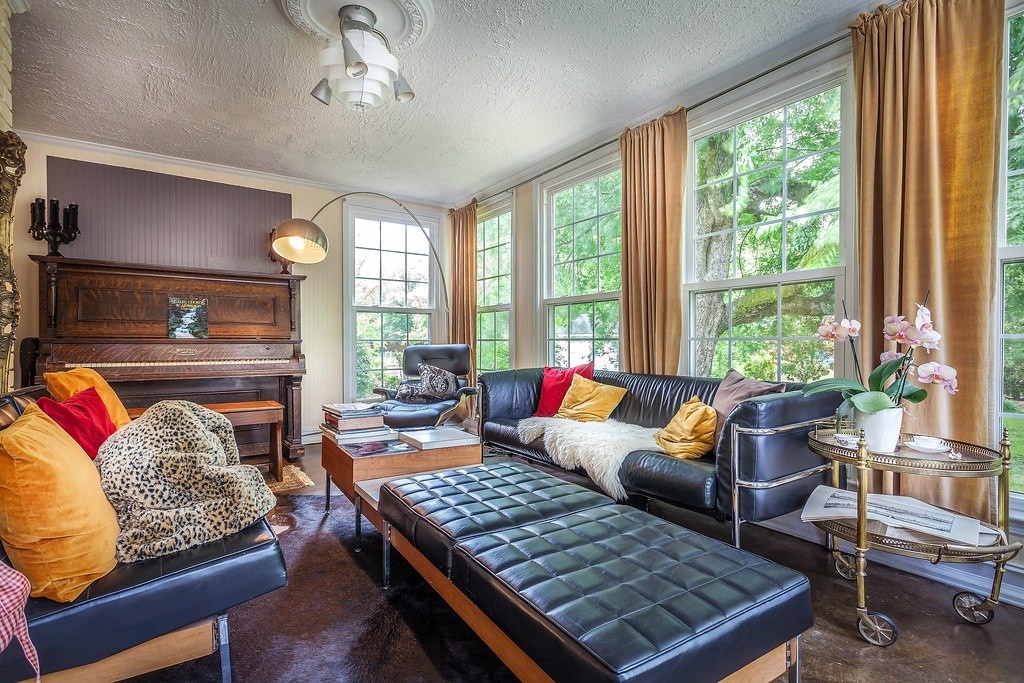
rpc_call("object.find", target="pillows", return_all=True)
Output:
[0,401,122,604]
[552,373,627,422]
[534,361,595,416]
[395,383,432,404]
[416,363,458,399]
[35,385,117,461]
[711,368,786,455]
[44,367,135,429]
[655,395,718,460]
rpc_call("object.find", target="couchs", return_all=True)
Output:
[476,366,843,551]
[0,377,288,683]
[373,344,470,428]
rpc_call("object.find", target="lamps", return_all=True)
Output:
[270,192,451,343]
[311,3,415,112]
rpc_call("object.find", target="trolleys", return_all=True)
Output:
[807,405,1024,648]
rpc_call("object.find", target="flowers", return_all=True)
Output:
[801,290,958,415]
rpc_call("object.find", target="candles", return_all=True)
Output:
[29,196,78,230]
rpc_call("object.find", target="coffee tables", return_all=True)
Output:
[321,430,813,683]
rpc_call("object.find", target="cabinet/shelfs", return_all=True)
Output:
[808,429,1022,649]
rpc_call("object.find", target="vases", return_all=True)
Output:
[854,406,903,454]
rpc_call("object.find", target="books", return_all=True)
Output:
[168,297,208,338]
[319,402,399,445]
[399,428,482,450]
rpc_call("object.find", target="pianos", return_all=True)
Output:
[28,254,308,463]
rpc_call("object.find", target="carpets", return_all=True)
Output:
[260,465,316,492]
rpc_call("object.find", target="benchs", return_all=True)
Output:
[126,400,285,481]
